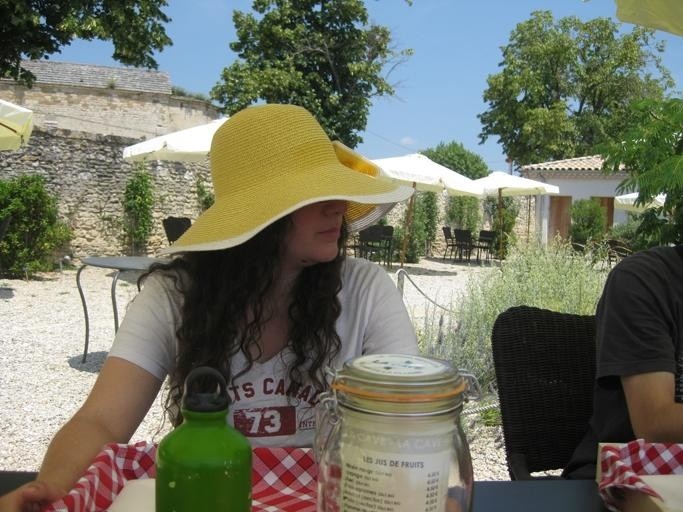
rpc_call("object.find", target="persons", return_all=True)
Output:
[1,104,421,512]
[559,196,683,478]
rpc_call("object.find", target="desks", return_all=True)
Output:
[75,256,173,364]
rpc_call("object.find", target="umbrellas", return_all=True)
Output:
[369,153,560,268]
[122,118,227,162]
[0,98,33,150]
[614,193,666,214]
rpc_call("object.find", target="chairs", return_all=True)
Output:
[441,225,497,264]
[490,303,597,480]
[343,225,398,269]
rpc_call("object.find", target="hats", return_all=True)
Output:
[166,103,415,256]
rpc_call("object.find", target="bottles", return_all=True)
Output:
[154,367,252,512]
[310,352,474,512]
